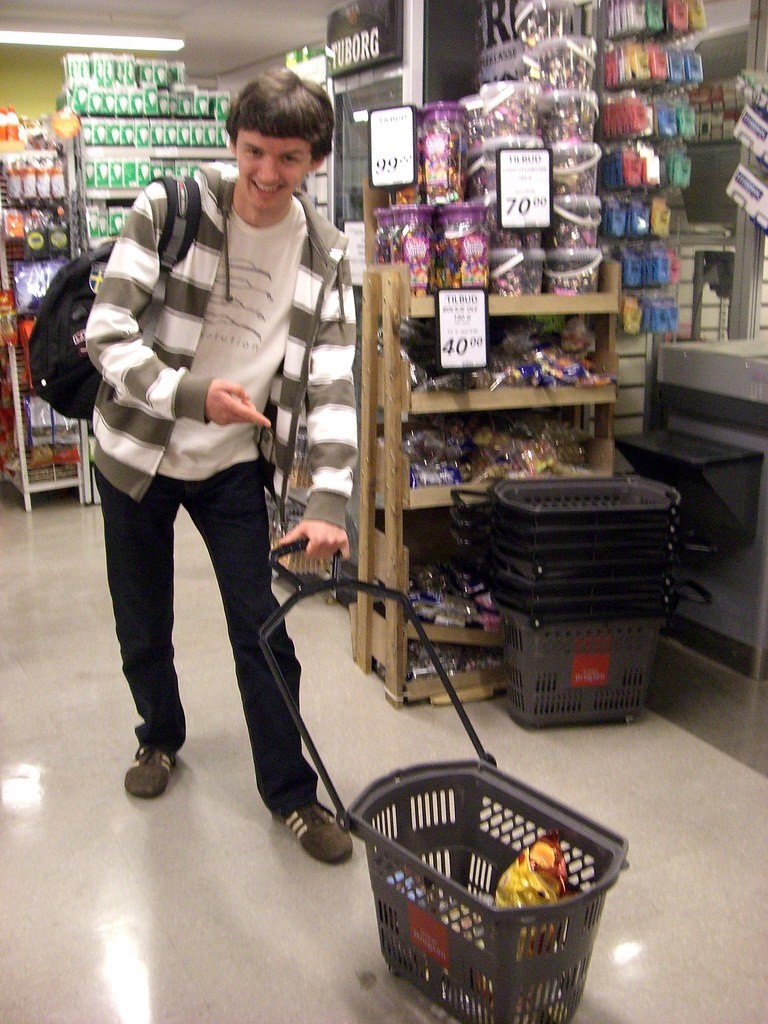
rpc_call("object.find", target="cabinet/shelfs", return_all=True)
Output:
[353,260,623,714]
[60,118,236,506]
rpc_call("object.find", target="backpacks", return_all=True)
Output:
[28,174,201,418]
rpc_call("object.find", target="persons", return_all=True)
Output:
[90,70,353,865]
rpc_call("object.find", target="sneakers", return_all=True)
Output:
[269,797,352,864]
[124,743,176,799]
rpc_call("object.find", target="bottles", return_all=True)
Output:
[0,105,19,141]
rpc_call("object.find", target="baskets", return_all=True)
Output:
[260,538,629,1024]
[448,474,682,731]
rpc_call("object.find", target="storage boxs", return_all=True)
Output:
[726,162,768,235]
[732,106,768,169]
